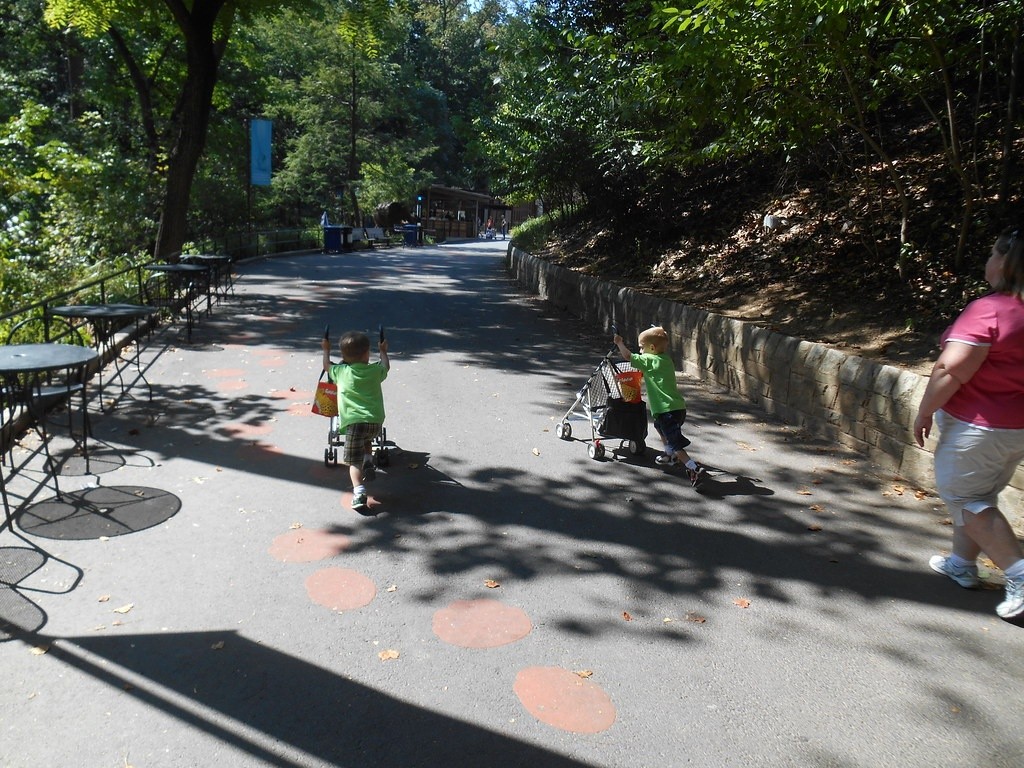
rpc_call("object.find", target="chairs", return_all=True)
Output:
[143,271,196,345]
[180,255,220,320]
[0,316,91,476]
[207,248,237,302]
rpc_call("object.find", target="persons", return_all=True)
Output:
[913,228,1024,620]
[320,330,389,508]
[477,215,506,240]
[614,327,705,487]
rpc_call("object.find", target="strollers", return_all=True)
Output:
[321,322,394,464]
[553,324,657,459]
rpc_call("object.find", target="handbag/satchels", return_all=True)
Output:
[606,356,642,404]
[596,369,649,442]
[311,361,338,418]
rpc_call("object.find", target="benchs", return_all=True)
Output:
[363,227,393,251]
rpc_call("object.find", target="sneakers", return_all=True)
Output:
[363,461,376,482]
[690,466,706,487]
[351,493,367,509]
[995,571,1024,619]
[654,452,678,466]
[929,555,979,589]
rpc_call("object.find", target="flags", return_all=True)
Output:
[250,119,273,186]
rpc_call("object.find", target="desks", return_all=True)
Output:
[180,255,234,307]
[141,263,210,330]
[0,343,100,503]
[49,302,158,412]
